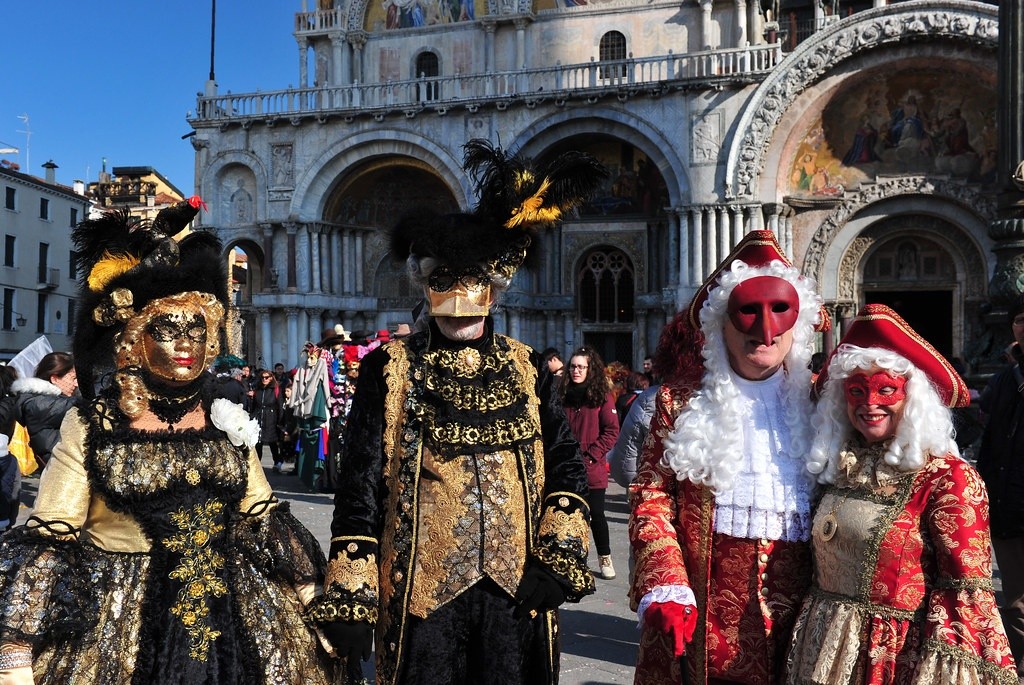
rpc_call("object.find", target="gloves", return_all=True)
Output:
[322,620,373,673]
[515,567,568,622]
[643,601,699,657]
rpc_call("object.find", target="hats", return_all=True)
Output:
[688,230,832,334]
[299,323,411,362]
[377,136,584,293]
[71,195,233,400]
[809,304,971,408]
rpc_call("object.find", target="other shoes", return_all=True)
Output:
[272,462,282,472]
[287,468,298,475]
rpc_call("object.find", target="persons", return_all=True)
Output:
[948,291,1024,677]
[0,195,333,685]
[0,351,92,537]
[626,229,827,685]
[215,362,294,472]
[322,141,592,685]
[786,302,1019,685]
[537,345,664,580]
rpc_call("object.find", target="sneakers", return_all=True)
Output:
[598,555,616,579]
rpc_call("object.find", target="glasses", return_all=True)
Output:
[261,375,271,380]
[569,365,588,371]
